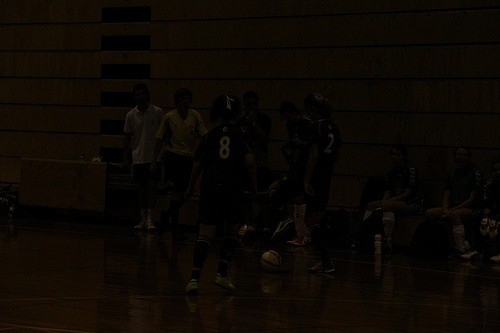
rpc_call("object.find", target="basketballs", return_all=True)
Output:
[261,249,281,270]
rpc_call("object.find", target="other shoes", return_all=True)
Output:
[145,218,156,229]
[161,210,169,230]
[488,256,500,263]
[134,221,144,228]
[448,252,458,259]
[172,231,184,240]
[308,262,336,273]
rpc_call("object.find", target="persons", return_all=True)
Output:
[303,92,341,274]
[424,144,485,261]
[123,84,164,231]
[481,160,500,264]
[183,93,260,293]
[151,88,209,241]
[229,89,315,253]
[367,144,421,256]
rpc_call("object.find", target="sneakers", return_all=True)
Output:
[288,236,312,246]
[213,273,235,289]
[185,278,200,292]
[271,216,294,241]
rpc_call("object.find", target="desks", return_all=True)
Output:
[18,156,110,213]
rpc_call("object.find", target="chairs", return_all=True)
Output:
[158,170,426,248]
